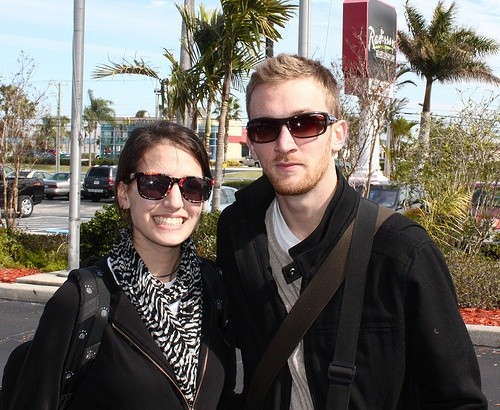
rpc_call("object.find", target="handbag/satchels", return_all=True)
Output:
[0,266,109,410]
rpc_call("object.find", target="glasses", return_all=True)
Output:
[127,172,212,203]
[247,112,338,143]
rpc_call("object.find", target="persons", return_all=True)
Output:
[7,120,232,409]
[215,52,489,410]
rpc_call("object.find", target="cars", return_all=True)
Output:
[41,171,89,202]
[204,185,239,215]
[6,169,55,200]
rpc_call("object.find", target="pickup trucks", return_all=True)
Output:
[0,167,45,218]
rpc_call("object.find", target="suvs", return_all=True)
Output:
[353,179,424,216]
[80,165,119,203]
[450,181,500,258]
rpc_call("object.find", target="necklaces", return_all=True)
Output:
[148,267,180,280]
